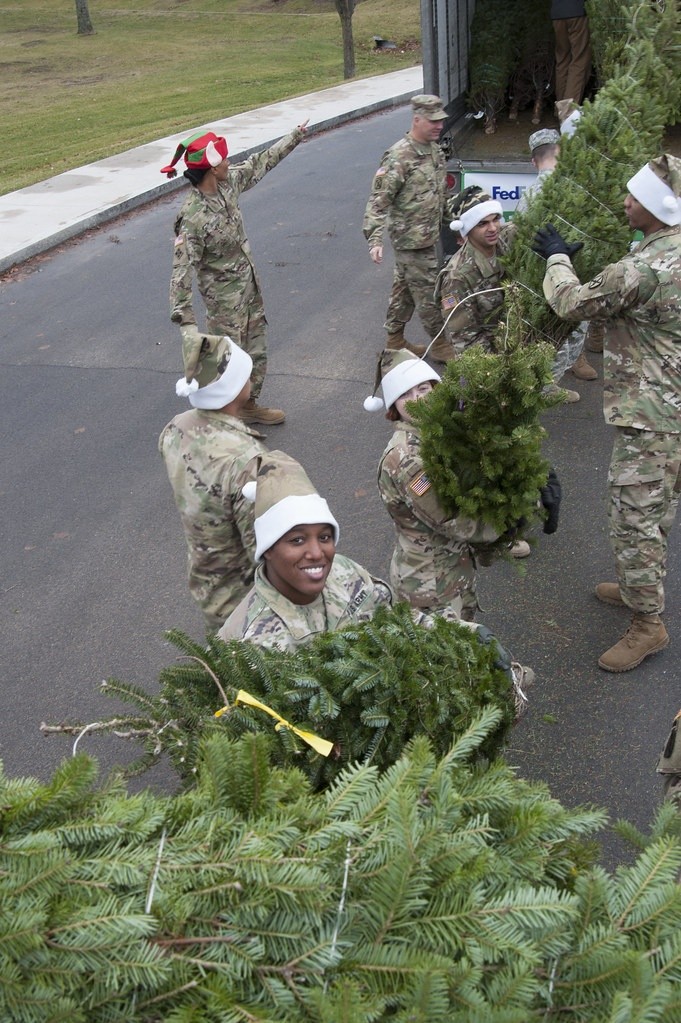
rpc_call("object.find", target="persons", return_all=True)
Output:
[532,154,681,672]
[513,131,603,403]
[363,95,457,363]
[205,449,512,671]
[550,0,592,127]
[162,117,312,425]
[363,348,562,623]
[159,333,266,635]
[433,186,531,558]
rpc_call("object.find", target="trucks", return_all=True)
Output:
[418,0,607,247]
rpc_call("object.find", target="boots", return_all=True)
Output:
[240,394,286,424]
[596,580,666,614]
[431,334,455,364]
[385,332,429,356]
[598,611,669,673]
[586,330,605,353]
[570,351,598,380]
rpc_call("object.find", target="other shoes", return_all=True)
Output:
[515,666,533,688]
[508,539,534,564]
[546,385,581,403]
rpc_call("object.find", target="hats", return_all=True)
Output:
[241,448,339,563]
[160,129,228,180]
[626,152,681,228]
[446,185,503,237]
[412,93,449,121]
[363,347,442,415]
[529,131,561,152]
[176,336,255,411]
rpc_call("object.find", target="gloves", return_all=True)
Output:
[475,626,513,671]
[530,221,584,259]
[540,470,563,537]
